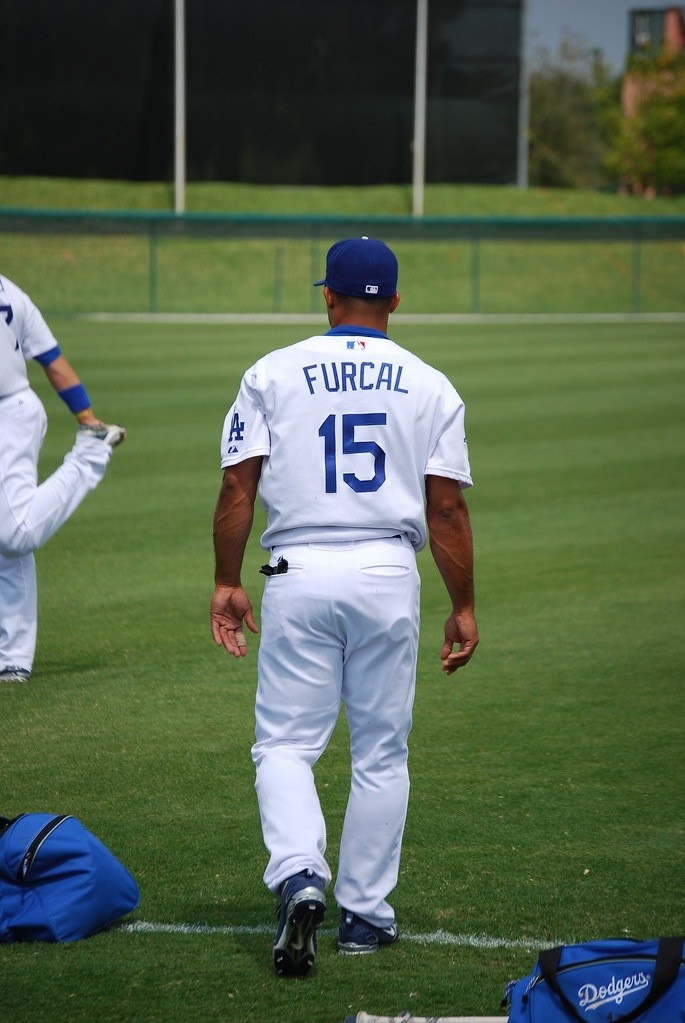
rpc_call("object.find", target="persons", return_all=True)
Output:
[213,237,480,979]
[0,274,127,683]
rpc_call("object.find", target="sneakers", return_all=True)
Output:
[80,423,126,447]
[272,869,328,978]
[333,907,400,955]
[0,666,31,684]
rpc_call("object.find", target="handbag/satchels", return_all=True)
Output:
[501,936,685,1023]
[0,812,140,945]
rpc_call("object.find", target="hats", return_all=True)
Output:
[313,235,398,298]
